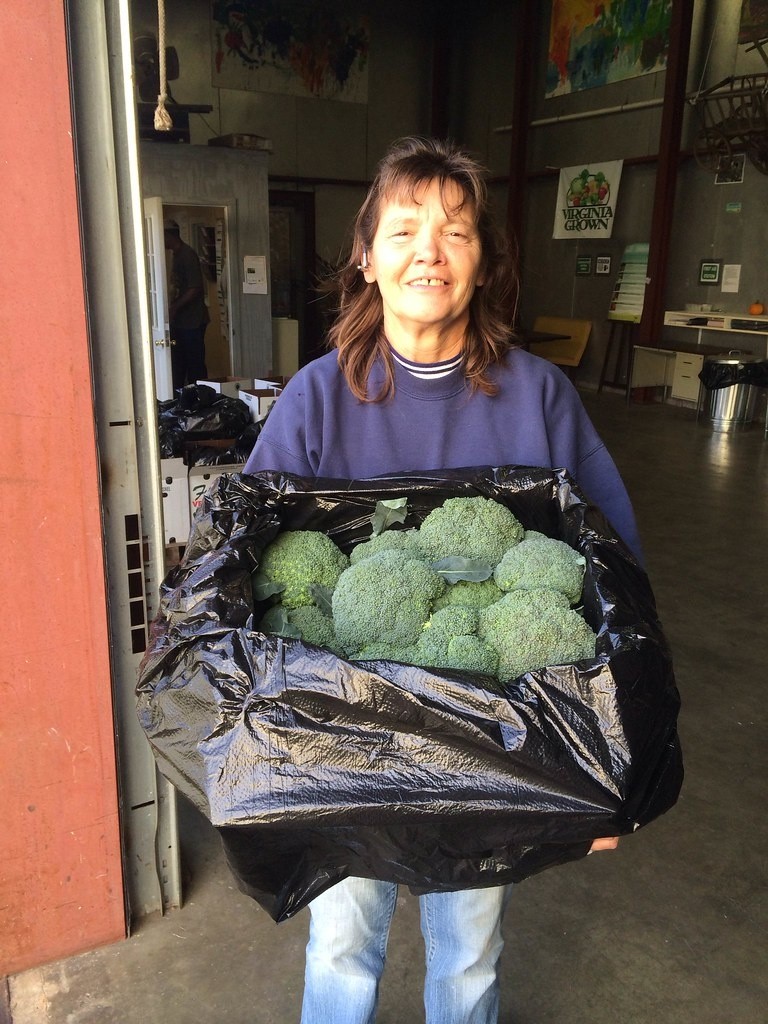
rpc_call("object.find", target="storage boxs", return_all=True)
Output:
[160,376,293,544]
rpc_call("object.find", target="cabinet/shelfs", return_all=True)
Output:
[627,345,707,425]
[664,311,768,335]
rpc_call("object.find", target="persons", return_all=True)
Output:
[163,220,211,383]
[241,140,645,1024]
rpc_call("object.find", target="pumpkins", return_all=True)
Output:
[748,300,764,315]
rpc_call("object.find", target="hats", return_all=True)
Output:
[163,219,180,229]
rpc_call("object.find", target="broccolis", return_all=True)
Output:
[249,496,598,679]
